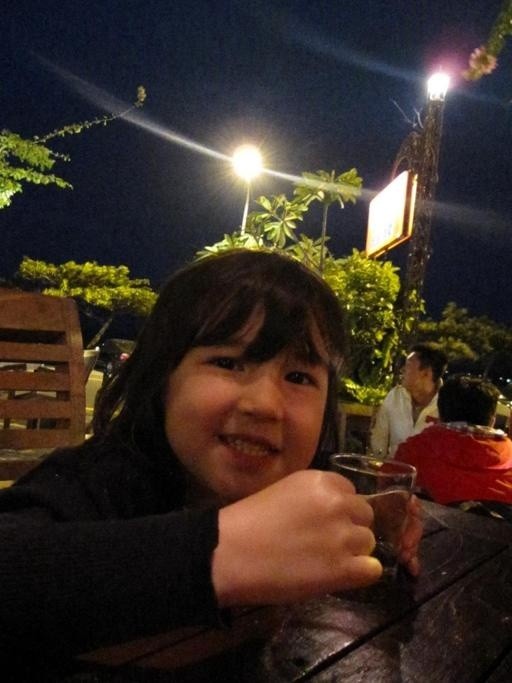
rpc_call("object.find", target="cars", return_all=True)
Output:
[328,452,419,588]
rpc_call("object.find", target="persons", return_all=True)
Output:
[367,344,448,459]
[378,377,512,504]
[2,250,425,655]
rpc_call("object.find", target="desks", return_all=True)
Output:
[231,141,262,235]
[366,70,452,345]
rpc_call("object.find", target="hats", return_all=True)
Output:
[2,309,130,442]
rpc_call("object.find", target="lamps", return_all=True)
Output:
[0,288,85,493]
